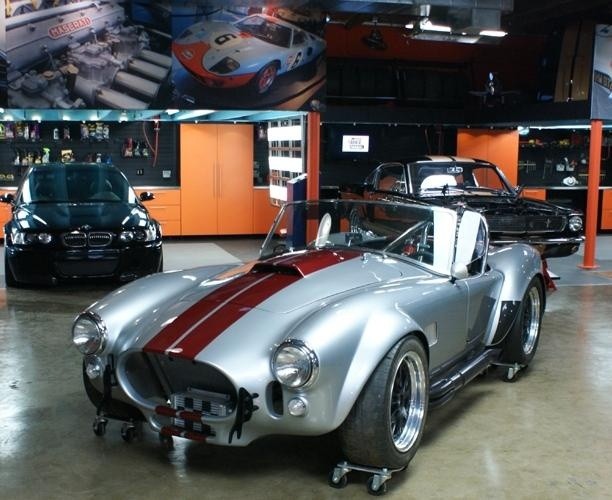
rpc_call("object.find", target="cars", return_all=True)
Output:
[1,159,166,291]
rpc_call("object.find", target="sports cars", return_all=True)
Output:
[73,187,555,491]
[171,13,327,97]
[341,152,586,263]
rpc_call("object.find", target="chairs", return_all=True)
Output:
[89,176,113,193]
[35,177,58,196]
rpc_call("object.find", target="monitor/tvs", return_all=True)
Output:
[340,133,371,154]
